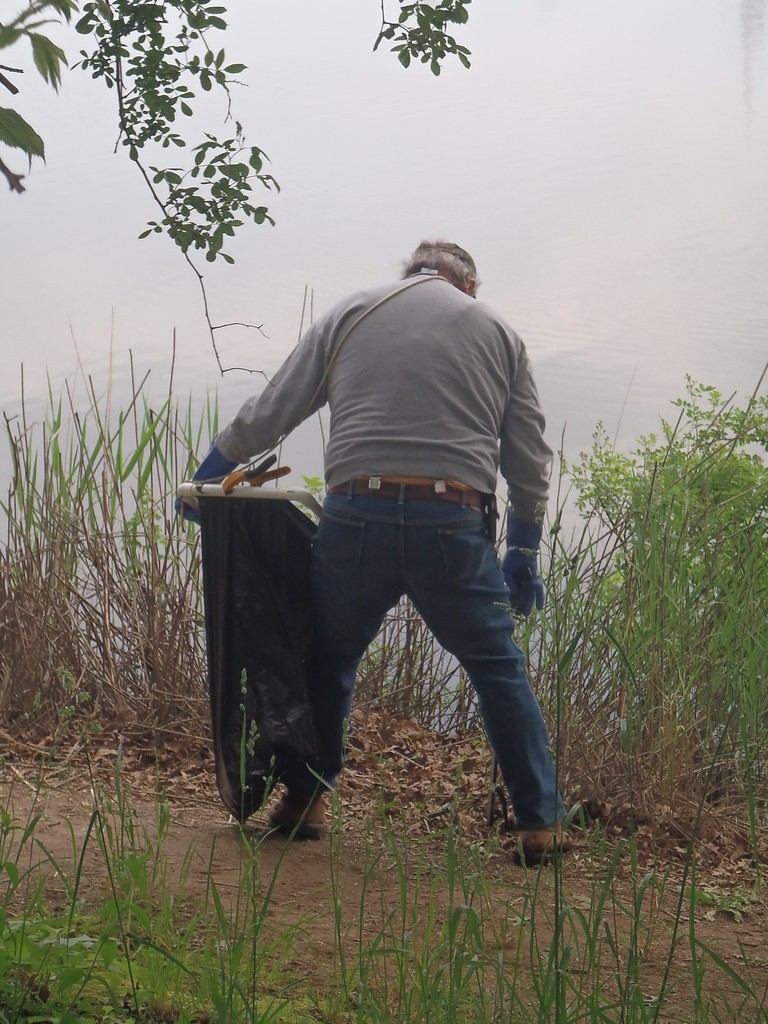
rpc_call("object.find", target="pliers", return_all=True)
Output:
[222,454,292,495]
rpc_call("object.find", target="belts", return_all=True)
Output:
[327,477,487,509]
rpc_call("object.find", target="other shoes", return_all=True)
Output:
[269,790,328,841]
[513,829,574,867]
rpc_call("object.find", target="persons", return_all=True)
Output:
[175,241,574,867]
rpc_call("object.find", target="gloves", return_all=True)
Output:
[500,510,545,618]
[174,437,240,528]
